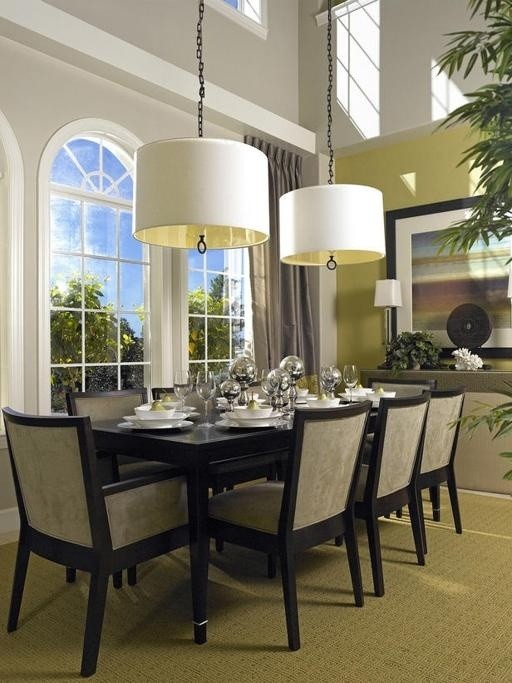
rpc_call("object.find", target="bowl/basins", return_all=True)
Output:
[345,388,371,395]
[220,411,286,426]
[135,406,175,417]
[123,414,191,428]
[298,389,309,396]
[306,397,341,408]
[155,400,181,409]
[250,393,259,399]
[217,398,229,401]
[368,392,397,400]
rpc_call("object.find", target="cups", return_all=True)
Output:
[343,364,357,401]
[261,369,279,407]
[174,371,193,433]
[196,372,216,428]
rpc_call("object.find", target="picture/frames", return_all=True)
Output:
[387,194,512,360]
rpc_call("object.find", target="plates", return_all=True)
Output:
[216,420,289,428]
[181,407,196,411]
[190,412,200,416]
[338,393,349,400]
[295,404,349,409]
[257,399,266,403]
[119,420,194,430]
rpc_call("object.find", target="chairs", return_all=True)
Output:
[65,389,148,585]
[267,391,430,600]
[368,386,465,534]
[211,401,370,649]
[367,378,436,518]
[6,404,185,675]
[152,387,175,403]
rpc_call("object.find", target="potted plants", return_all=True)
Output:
[451,348,483,370]
[377,331,441,370]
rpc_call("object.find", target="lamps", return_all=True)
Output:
[131,0,268,249]
[278,1,386,271]
[371,280,400,369]
